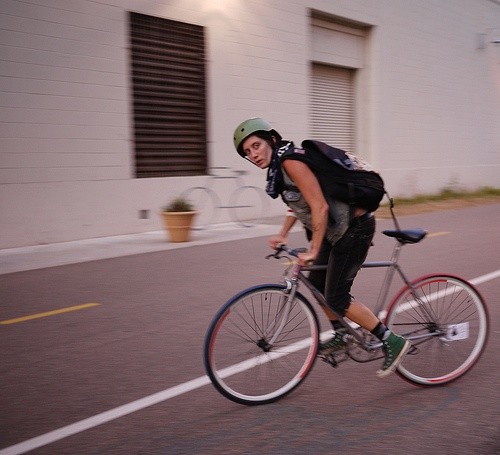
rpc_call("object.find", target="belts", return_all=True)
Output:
[353,212,375,224]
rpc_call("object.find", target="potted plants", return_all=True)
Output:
[158,197,201,244]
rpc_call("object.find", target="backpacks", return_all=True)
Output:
[280,140,386,227]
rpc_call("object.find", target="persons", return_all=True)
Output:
[233,117,412,377]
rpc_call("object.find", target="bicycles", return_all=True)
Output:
[203,228,492,406]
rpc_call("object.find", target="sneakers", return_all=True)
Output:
[376,330,413,379]
[318,329,353,354]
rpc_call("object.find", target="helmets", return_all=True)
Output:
[233,117,283,158]
